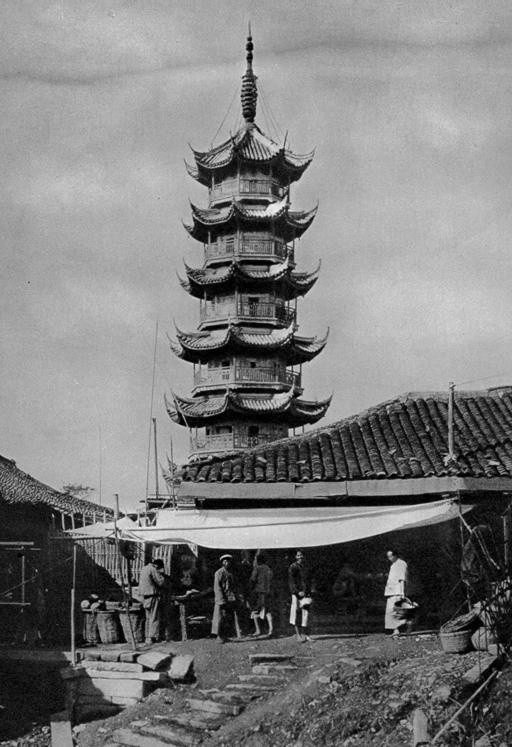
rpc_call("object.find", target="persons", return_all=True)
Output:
[333,559,360,598]
[136,549,316,643]
[383,547,414,636]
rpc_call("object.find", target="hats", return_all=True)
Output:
[219,553,232,561]
